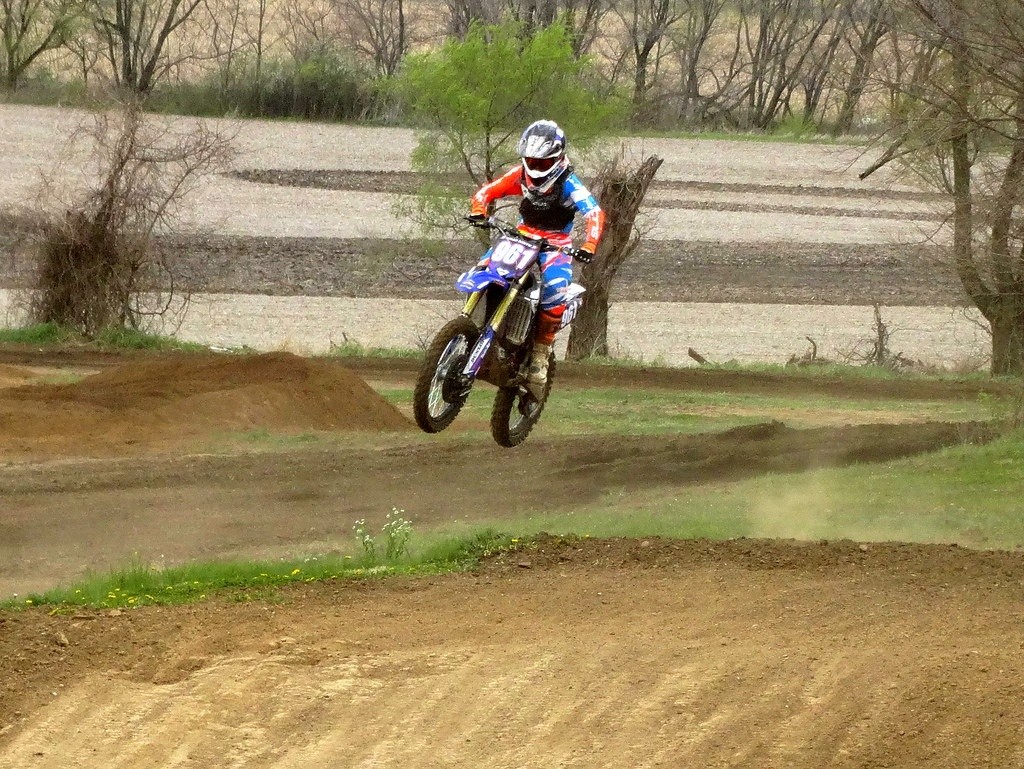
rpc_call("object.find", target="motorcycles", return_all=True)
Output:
[411,214,596,451]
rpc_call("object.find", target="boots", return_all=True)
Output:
[528,336,556,383]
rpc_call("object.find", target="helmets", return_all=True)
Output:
[518,120,570,195]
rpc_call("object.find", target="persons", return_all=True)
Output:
[468,119,606,383]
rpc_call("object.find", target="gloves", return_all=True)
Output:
[574,247,592,265]
[469,213,486,228]
